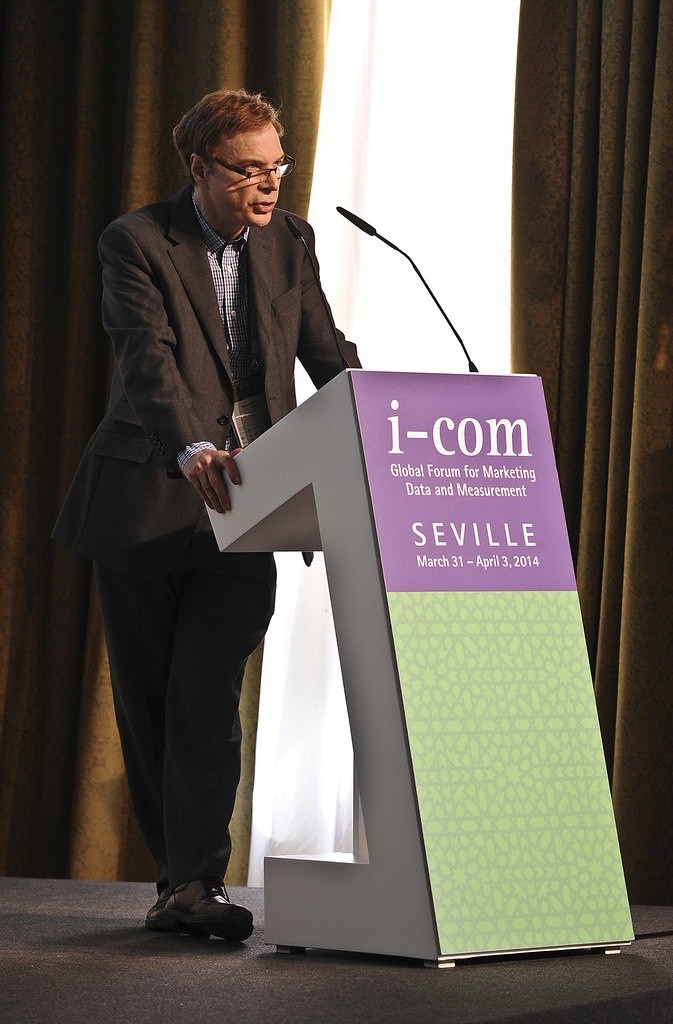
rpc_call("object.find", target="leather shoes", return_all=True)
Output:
[164,879,254,943]
[144,890,171,930]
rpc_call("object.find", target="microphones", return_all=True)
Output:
[336,205,479,373]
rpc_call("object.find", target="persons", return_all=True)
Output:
[51,90,366,943]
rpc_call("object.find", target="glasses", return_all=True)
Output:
[204,153,296,184]
